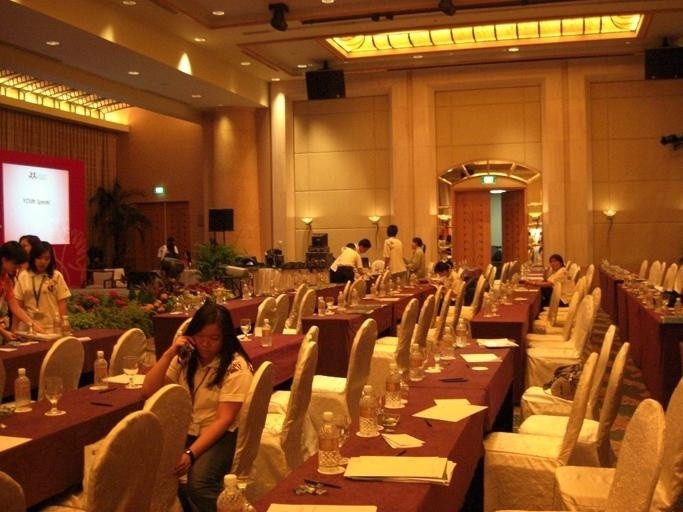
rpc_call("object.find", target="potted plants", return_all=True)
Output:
[88,179,153,281]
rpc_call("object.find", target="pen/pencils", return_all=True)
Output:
[304,479,342,488]
[98,387,117,394]
[89,401,113,406]
[394,448,406,456]
[424,418,432,427]
[465,363,470,368]
[439,378,467,382]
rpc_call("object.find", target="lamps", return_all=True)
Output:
[323,13,645,60]
[529,211,542,231]
[368,217,382,252]
[602,209,617,243]
[1,67,136,123]
[298,218,314,244]
[437,214,452,227]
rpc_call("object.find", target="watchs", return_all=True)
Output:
[184,449,195,462]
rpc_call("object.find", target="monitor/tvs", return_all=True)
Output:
[3,161,70,248]
[208,208,233,232]
[310,232,327,247]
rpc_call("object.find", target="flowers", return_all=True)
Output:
[140,281,232,316]
[65,290,158,337]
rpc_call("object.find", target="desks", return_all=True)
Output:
[605,275,648,323]
[407,337,515,436]
[513,270,546,293]
[357,282,442,339]
[469,301,532,386]
[0,329,126,402]
[502,292,542,336]
[252,386,490,512]
[299,301,393,379]
[627,285,682,410]
[151,278,375,363]
[252,269,331,296]
[1,333,307,509]
[599,266,633,313]
[615,281,648,339]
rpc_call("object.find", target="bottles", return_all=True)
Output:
[351,288,359,307]
[385,362,401,408]
[483,292,491,316]
[507,280,511,290]
[396,277,402,291]
[388,279,393,291]
[94,351,108,386]
[379,281,385,297]
[14,367,30,408]
[261,319,271,347]
[442,326,453,357]
[243,283,249,300]
[501,283,507,299]
[512,273,521,287]
[317,411,341,473]
[338,291,346,311]
[62,315,71,337]
[53,313,61,335]
[410,273,417,286]
[216,473,245,512]
[370,283,376,297]
[270,280,275,297]
[318,296,325,316]
[455,318,468,348]
[359,386,376,435]
[409,343,424,383]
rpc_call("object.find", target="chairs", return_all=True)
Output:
[389,307,437,350]
[38,336,85,402]
[374,274,383,293]
[445,281,466,337]
[263,326,319,456]
[384,270,391,290]
[501,262,509,285]
[488,267,497,283]
[532,282,562,334]
[495,261,518,285]
[254,297,276,335]
[486,263,492,276]
[554,372,682,511]
[266,249,282,268]
[39,410,164,512]
[482,353,598,510]
[517,340,631,467]
[255,342,317,488]
[521,323,616,418]
[537,264,595,319]
[495,398,666,512]
[290,283,308,322]
[309,319,377,435]
[568,263,577,276]
[376,295,436,355]
[662,262,678,292]
[1,471,25,511]
[276,294,289,331]
[61,382,194,512]
[446,276,486,320]
[176,359,276,511]
[546,275,588,335]
[566,261,572,268]
[539,278,583,310]
[297,289,316,332]
[570,268,582,280]
[527,287,602,349]
[371,260,385,273]
[656,261,665,288]
[648,260,660,284]
[396,285,440,327]
[369,297,418,386]
[672,265,683,292]
[525,292,579,342]
[343,280,351,302]
[425,288,453,344]
[108,328,147,378]
[449,274,483,311]
[348,280,367,305]
[639,259,648,279]
[525,294,594,386]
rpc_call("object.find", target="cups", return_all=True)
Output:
[325,297,335,314]
[240,318,252,341]
[43,376,66,416]
[122,356,143,389]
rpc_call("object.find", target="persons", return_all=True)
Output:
[142,301,254,512]
[445,262,452,272]
[328,239,372,283]
[383,224,407,282]
[19,235,41,271]
[430,261,463,298]
[524,255,576,306]
[403,237,426,278]
[183,250,192,267]
[0,241,47,345]
[337,243,363,283]
[157,237,178,261]
[11,242,72,333]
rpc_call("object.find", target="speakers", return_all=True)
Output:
[306,69,345,101]
[645,46,682,80]
